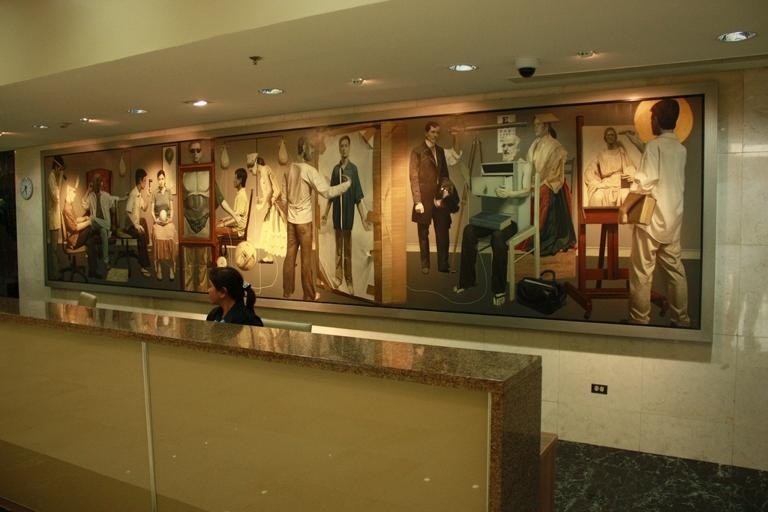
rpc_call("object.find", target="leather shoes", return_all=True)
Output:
[169,279,175,281]
[157,278,163,281]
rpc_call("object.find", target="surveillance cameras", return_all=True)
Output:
[516,58,538,78]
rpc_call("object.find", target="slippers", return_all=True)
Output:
[453,283,465,296]
[491,292,507,306]
[140,271,152,278]
[105,264,111,270]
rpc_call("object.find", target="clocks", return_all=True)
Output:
[20,176,32,201]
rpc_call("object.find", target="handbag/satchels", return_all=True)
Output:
[517,270,569,314]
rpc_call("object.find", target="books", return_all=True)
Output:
[625,194,657,225]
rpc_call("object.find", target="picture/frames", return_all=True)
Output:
[38,77,717,348]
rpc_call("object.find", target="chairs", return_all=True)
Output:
[216,188,256,255]
[112,194,141,277]
[58,211,89,284]
[470,175,543,306]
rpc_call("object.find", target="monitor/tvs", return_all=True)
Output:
[77,292,97,306]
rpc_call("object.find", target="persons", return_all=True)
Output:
[407,120,458,274]
[204,266,265,326]
[581,126,640,209]
[613,97,693,329]
[512,112,577,258]
[452,134,533,307]
